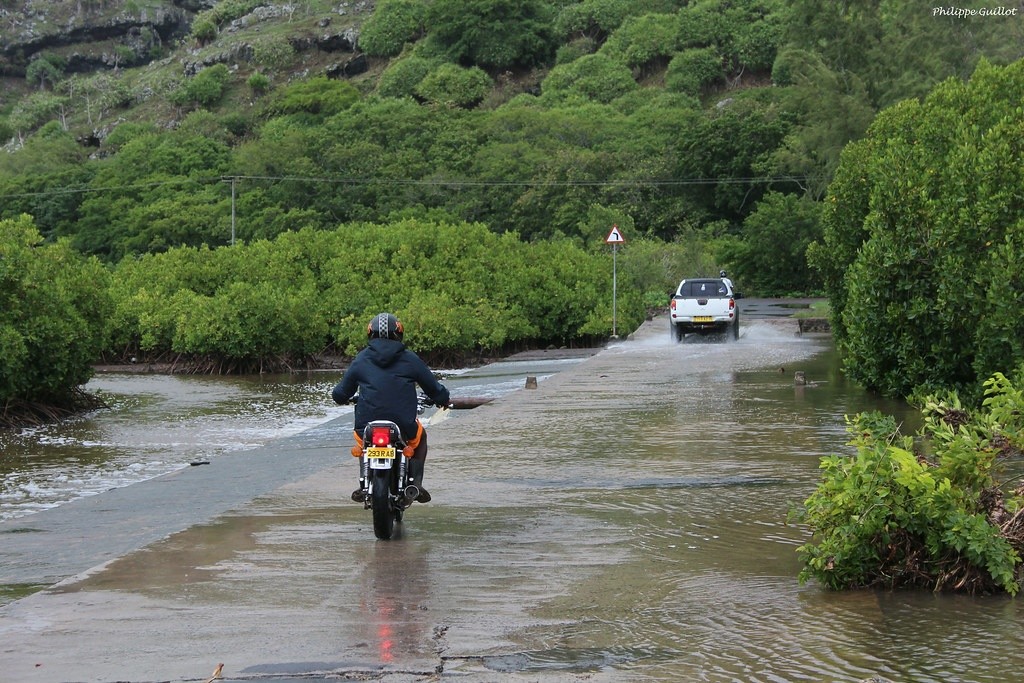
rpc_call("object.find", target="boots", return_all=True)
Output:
[351,457,369,502]
[407,458,431,503]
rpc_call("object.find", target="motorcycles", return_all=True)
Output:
[346,391,453,540]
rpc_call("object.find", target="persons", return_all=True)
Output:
[332,313,450,503]
[720,270,733,289]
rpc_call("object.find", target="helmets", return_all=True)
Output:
[366,313,404,342]
[720,270,727,277]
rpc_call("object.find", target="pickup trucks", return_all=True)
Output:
[669,278,739,342]
